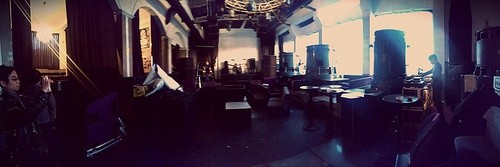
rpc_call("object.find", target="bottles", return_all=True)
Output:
[243,96,247,107]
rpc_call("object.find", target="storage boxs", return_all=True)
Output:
[226,102,251,129]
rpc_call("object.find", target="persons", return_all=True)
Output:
[419,54,444,113]
[0,63,55,167]
[23,68,57,167]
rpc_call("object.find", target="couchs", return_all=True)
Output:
[268,87,290,118]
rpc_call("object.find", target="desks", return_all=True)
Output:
[300,85,320,131]
[341,92,365,145]
[319,88,344,137]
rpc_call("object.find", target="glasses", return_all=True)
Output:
[8,76,20,83]
